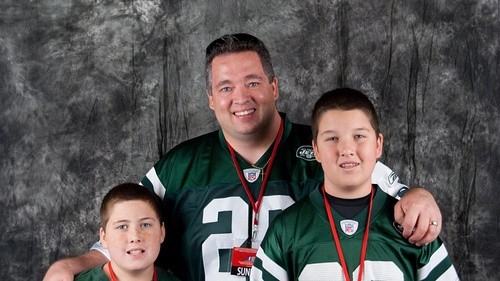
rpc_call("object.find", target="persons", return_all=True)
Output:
[73,182,180,281]
[41,32,442,281]
[247,88,461,281]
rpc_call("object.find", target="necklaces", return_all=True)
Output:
[250,158,270,182]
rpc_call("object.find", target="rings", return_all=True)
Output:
[429,221,438,226]
